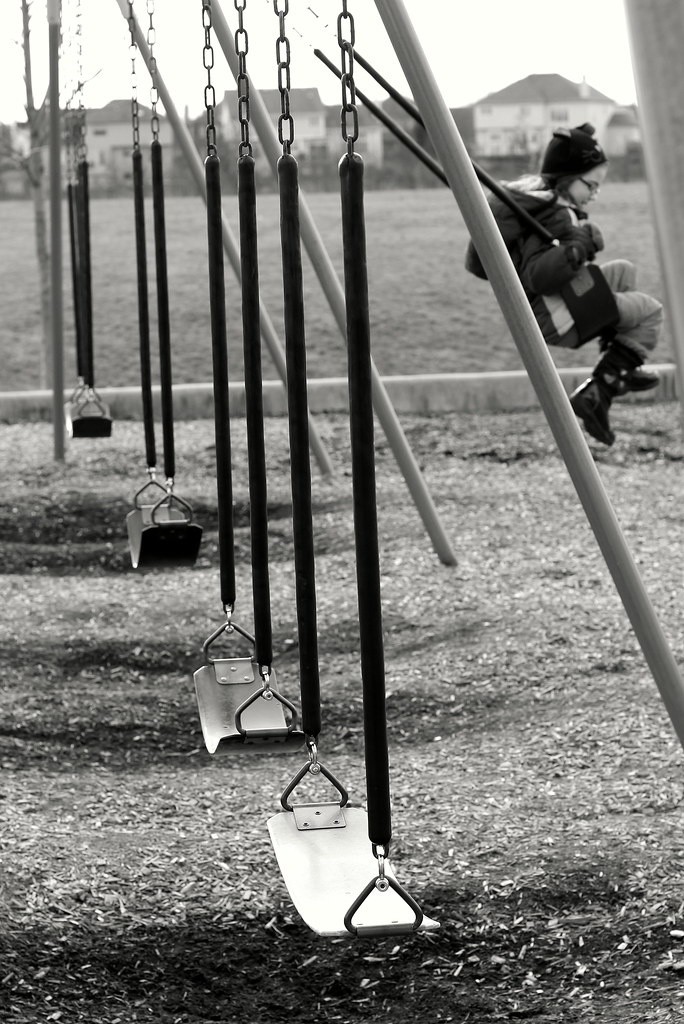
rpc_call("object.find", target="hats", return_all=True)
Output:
[540,123,607,194]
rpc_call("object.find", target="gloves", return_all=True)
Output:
[566,223,604,260]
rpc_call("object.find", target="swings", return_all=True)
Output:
[123,0,206,575]
[260,0,631,353]
[49,0,116,440]
[261,0,448,944]
[191,0,309,764]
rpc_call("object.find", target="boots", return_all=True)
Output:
[624,369,659,392]
[569,343,644,446]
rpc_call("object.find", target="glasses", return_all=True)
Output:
[579,177,600,194]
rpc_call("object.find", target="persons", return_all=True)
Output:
[462,122,664,446]
